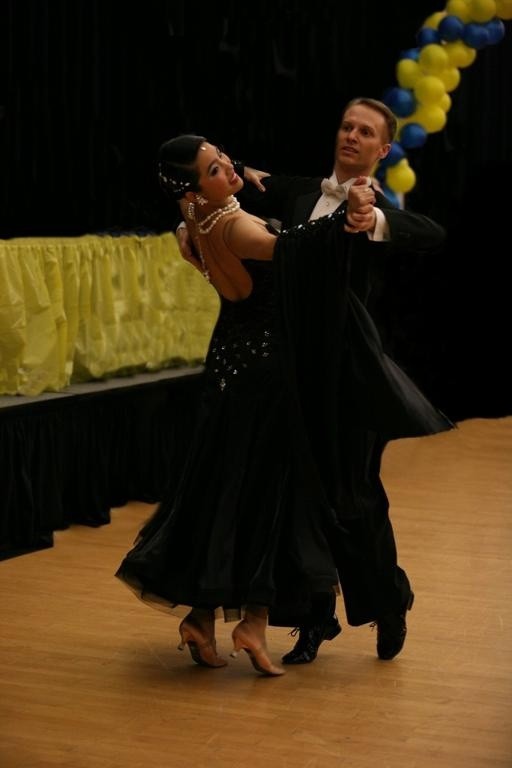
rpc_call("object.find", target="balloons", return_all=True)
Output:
[373,1,511,213]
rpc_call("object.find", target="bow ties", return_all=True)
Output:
[320,178,348,200]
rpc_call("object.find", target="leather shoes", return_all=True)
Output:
[377,576,414,660]
[282,612,342,664]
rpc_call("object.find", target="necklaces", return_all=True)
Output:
[186,196,242,281]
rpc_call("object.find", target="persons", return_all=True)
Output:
[168,96,461,667]
[114,132,379,675]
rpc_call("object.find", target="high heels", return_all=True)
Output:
[178,616,227,667]
[230,621,285,676]
